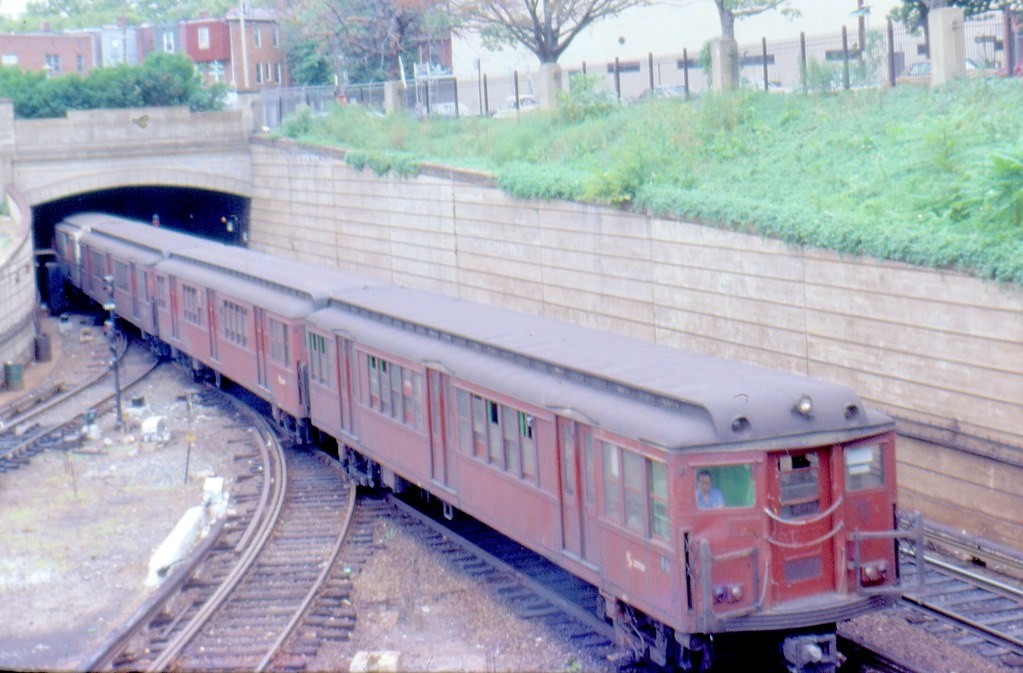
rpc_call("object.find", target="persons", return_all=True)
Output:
[693,469,728,511]
[788,455,823,516]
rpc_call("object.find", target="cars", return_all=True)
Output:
[277,49,1005,118]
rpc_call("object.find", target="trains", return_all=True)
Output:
[50,210,927,673]
